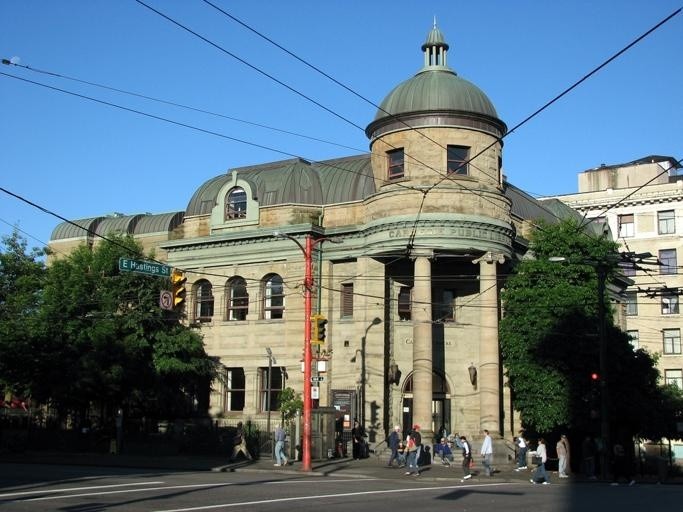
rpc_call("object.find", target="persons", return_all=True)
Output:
[458,436,472,483]
[480,429,494,477]
[352,420,361,463]
[334,411,345,458]
[528,437,552,485]
[389,425,463,479]
[229,419,254,464]
[609,434,637,486]
[580,432,598,482]
[111,409,125,454]
[274,422,288,467]
[512,434,528,472]
[554,433,569,480]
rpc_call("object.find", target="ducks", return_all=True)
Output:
[359,438,369,458]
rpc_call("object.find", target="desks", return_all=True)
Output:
[547,251,651,486]
[271,228,342,471]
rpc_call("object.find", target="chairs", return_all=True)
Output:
[383,464,420,478]
[273,460,287,467]
[462,466,569,485]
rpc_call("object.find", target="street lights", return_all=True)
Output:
[531,456,542,465]
[233,437,241,444]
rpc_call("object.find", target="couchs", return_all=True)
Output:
[591,369,599,386]
[313,313,326,346]
[171,269,187,312]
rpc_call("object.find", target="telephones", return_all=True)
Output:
[118,257,171,278]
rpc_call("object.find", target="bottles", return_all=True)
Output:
[394,425,400,429]
[413,424,419,429]
